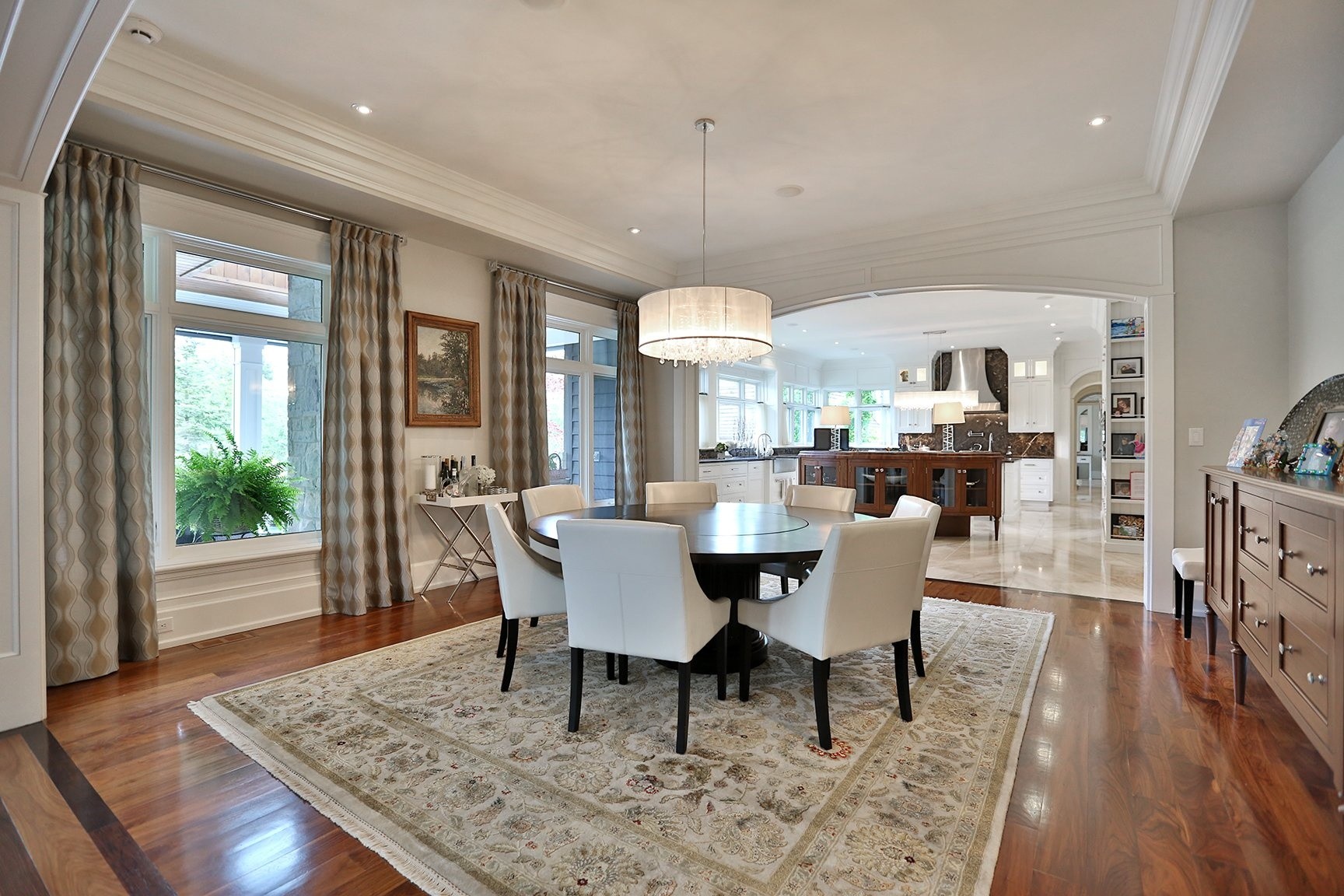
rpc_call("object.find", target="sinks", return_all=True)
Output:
[758,456,797,474]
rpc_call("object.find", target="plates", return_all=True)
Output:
[480,485,508,495]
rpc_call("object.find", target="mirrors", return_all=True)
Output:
[1080,427,1088,452]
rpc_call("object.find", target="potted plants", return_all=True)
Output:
[173,426,310,544]
[714,442,728,459]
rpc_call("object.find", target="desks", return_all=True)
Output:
[527,501,879,675]
[412,492,518,603]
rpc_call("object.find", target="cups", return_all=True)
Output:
[421,455,441,495]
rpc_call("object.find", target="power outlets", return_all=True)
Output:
[158,617,175,633]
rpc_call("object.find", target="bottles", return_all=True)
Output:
[438,455,479,498]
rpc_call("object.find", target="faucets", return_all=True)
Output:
[757,433,772,457]
[988,432,994,452]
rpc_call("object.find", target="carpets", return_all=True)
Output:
[186,572,1055,896]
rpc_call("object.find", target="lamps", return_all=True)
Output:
[819,406,851,452]
[893,326,980,410]
[638,117,773,368]
[931,402,966,453]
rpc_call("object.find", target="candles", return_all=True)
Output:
[425,464,436,489]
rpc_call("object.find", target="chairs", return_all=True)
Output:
[484,481,942,754]
[1171,547,1204,640]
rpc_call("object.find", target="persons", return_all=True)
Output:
[1118,437,1130,454]
[1116,484,1129,495]
[1121,364,1135,373]
[1114,399,1130,415]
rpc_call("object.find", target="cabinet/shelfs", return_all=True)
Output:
[696,357,1099,539]
[1198,467,1344,812]
[1103,300,1145,554]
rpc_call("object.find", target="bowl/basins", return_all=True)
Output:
[862,474,907,483]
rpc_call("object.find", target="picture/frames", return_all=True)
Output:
[405,309,481,428]
[1294,408,1344,476]
[1110,318,1145,541]
[1227,418,1268,467]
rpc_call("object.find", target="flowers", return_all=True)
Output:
[475,464,497,485]
